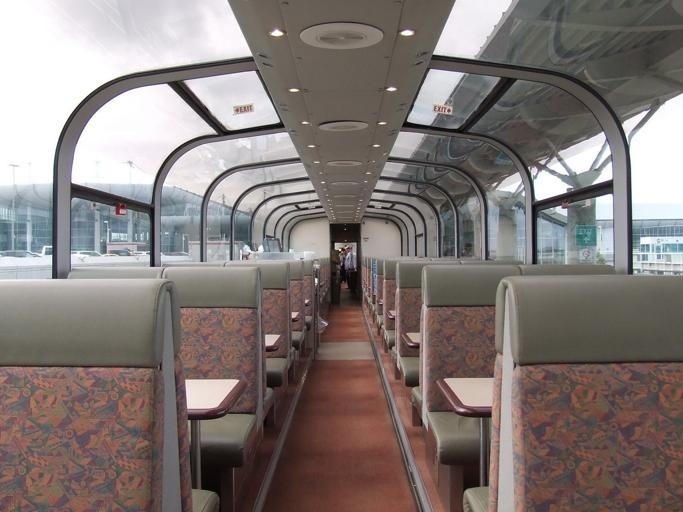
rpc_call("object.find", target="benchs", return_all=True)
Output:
[360,252,682,512]
[0,249,329,509]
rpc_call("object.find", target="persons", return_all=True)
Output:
[462,243,473,256]
[339,246,353,283]
